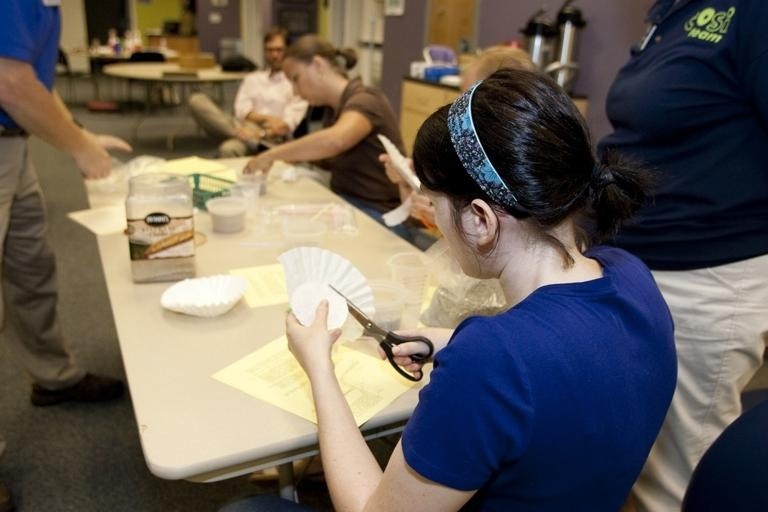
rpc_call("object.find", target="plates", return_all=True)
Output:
[161,274,248,317]
[279,247,375,346]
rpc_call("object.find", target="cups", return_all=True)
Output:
[387,252,432,316]
[232,184,259,219]
[369,283,409,334]
[205,197,245,234]
[236,169,262,191]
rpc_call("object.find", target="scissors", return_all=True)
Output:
[328,284,433,381]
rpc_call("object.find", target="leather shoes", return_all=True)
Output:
[31,372,126,408]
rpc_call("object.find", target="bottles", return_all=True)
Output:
[522,5,582,97]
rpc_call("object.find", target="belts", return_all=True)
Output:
[0,126,25,137]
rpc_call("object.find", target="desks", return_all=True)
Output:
[397,76,589,159]
[84,157,487,504]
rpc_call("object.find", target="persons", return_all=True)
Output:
[184,25,312,161]
[241,33,408,239]
[216,63,675,509]
[378,42,541,236]
[680,393,766,512]
[0,1,135,409]
[578,2,766,510]
